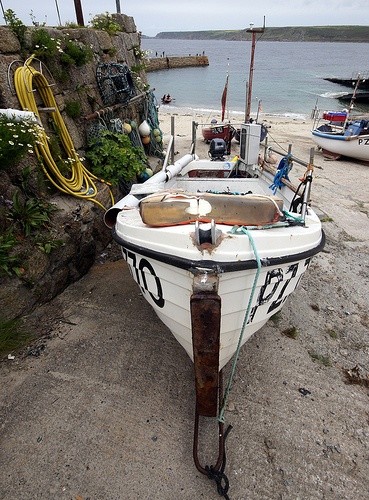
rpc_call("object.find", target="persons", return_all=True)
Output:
[155,51,207,69]
[162,94,171,101]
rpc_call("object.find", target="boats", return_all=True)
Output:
[235,123,271,142]
[102,134,327,419]
[309,107,369,164]
[200,121,236,142]
[161,97,175,103]
